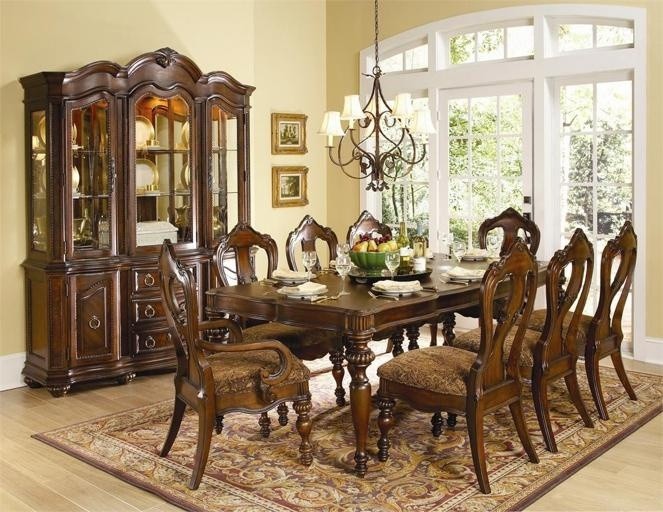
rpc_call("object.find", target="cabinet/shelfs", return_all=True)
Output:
[18,46,256,397]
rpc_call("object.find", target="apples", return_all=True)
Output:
[378,241,397,252]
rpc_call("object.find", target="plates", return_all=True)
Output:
[271,274,316,284]
[180,163,190,190]
[134,116,155,148]
[439,271,482,282]
[376,290,420,297]
[181,122,190,146]
[39,163,80,195]
[462,255,485,261]
[37,115,76,147]
[134,158,159,194]
[277,289,328,298]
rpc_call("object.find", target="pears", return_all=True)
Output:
[353,240,378,252]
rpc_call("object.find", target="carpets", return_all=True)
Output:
[30,336,663,512]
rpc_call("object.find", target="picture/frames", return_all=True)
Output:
[272,113,309,155]
[272,165,309,207]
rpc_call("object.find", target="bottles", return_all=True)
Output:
[97,214,109,250]
[411,220,427,256]
[79,208,91,236]
[396,222,410,274]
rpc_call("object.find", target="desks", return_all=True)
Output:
[206,257,565,477]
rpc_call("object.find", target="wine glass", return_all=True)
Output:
[335,243,350,257]
[334,257,352,295]
[436,230,453,262]
[383,251,400,281]
[301,250,318,279]
[451,242,465,264]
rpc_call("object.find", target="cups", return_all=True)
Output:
[31,135,39,150]
[412,257,425,273]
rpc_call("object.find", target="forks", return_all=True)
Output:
[439,278,468,285]
[367,290,399,302]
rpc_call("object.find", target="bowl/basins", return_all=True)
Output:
[349,251,398,273]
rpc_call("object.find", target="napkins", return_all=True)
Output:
[373,279,421,292]
[272,267,316,279]
[282,280,327,294]
[446,266,485,279]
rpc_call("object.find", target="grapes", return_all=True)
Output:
[355,232,391,245]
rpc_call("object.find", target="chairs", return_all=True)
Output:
[445,227,596,454]
[157,238,313,492]
[377,237,540,493]
[457,208,541,321]
[287,215,407,409]
[210,222,346,427]
[513,219,639,421]
[347,212,457,350]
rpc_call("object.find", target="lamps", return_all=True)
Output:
[317,1,439,191]
